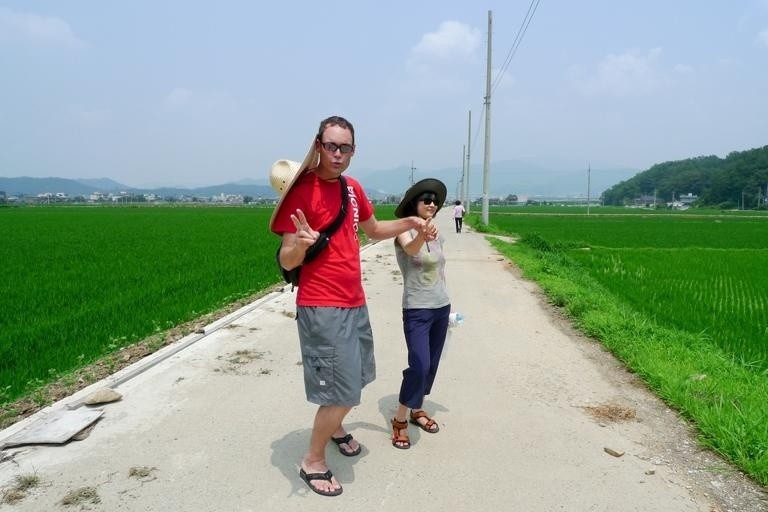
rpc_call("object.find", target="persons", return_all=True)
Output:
[452,200,467,234]
[272,114,439,496]
[388,177,453,450]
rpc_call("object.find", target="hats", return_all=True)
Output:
[394,178,447,218]
[269,133,319,237]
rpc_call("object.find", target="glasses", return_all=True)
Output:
[320,142,353,153]
[419,198,440,205]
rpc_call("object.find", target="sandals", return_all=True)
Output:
[391,416,410,449]
[409,407,439,433]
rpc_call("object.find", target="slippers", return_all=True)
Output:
[330,433,360,456]
[299,469,343,496]
